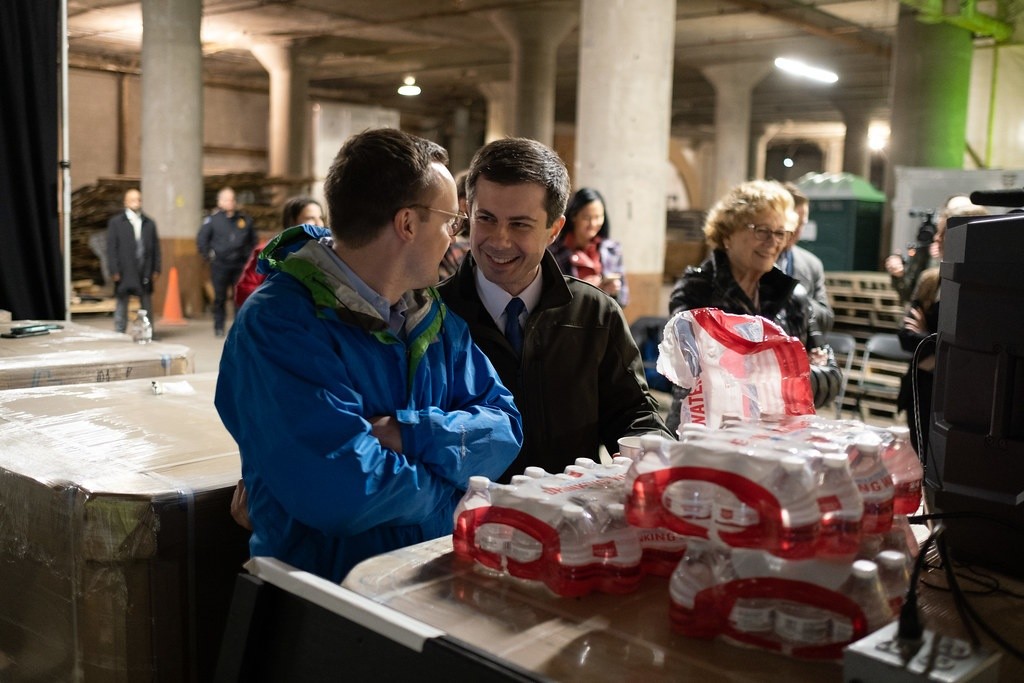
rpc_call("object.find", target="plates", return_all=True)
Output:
[446,417,922,659]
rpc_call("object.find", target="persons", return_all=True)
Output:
[886,196,987,466]
[195,127,676,593]
[668,179,843,437]
[105,189,163,338]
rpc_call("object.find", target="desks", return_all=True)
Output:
[339,485,1023,682]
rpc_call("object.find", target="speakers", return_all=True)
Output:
[914,212,1024,583]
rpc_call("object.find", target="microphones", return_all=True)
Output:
[970,189,1024,209]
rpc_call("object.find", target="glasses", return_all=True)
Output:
[409,203,468,236]
[744,223,793,244]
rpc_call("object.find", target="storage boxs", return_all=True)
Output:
[0,319,242,683]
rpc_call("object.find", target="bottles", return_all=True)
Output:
[132,310,152,345]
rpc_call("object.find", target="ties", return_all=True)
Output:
[504,297,524,358]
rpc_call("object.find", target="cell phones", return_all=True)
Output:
[600,274,621,286]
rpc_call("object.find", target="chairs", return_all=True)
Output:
[823,333,914,424]
[631,318,669,347]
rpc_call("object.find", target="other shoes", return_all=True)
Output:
[215,330,224,338]
